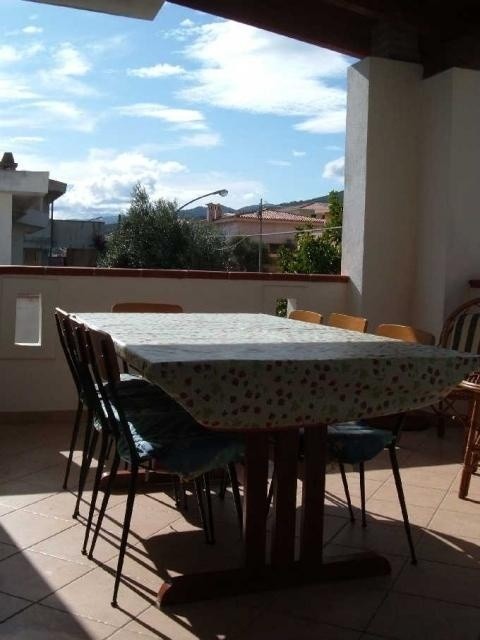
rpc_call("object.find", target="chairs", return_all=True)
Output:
[434,298,480,498]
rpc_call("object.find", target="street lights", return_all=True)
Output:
[175,189,228,220]
